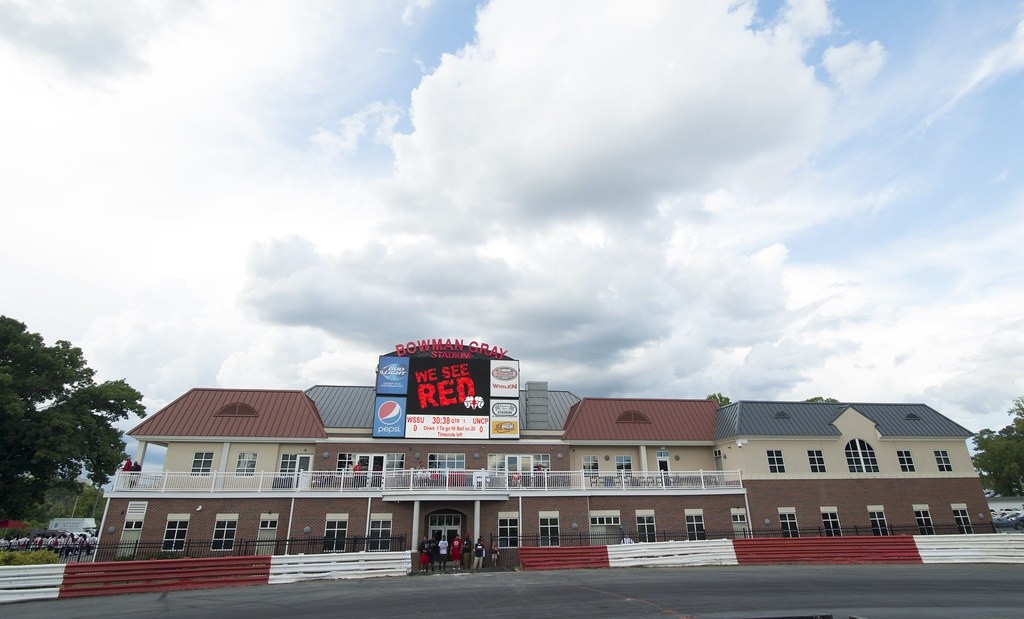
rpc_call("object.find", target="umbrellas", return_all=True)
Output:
[0,519,27,528]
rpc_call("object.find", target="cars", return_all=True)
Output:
[999,507,1023,517]
[30,530,77,542]
[73,532,97,541]
[985,491,991,498]
[990,508,999,518]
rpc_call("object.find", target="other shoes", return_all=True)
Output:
[439,568,446,571]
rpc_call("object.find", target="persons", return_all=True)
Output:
[620,535,634,544]
[129,460,142,488]
[419,535,438,573]
[450,534,463,571]
[490,540,499,568]
[351,460,363,488]
[536,463,543,487]
[119,457,132,471]
[416,461,427,486]
[0,529,99,559]
[438,535,448,573]
[472,536,485,570]
[462,534,473,570]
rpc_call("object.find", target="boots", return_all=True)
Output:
[426,568,429,573]
[432,567,435,571]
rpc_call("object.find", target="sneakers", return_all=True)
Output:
[419,569,426,573]
[453,567,460,570]
